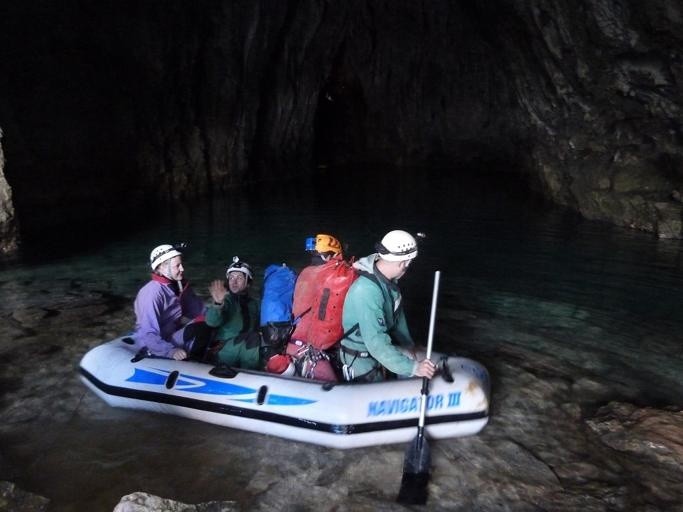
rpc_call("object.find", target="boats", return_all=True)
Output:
[77,333,491,450]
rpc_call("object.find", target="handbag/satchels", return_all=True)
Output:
[260,264,297,346]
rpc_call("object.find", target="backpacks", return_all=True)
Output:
[306,255,386,350]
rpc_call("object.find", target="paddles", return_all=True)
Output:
[395,271,442,505]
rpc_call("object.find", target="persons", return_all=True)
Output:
[286,235,358,384]
[260,255,301,347]
[339,230,438,382]
[205,258,275,370]
[134,244,211,360]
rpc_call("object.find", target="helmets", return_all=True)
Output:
[150,244,183,270]
[378,229,418,262]
[315,234,343,255]
[226,261,255,280]
